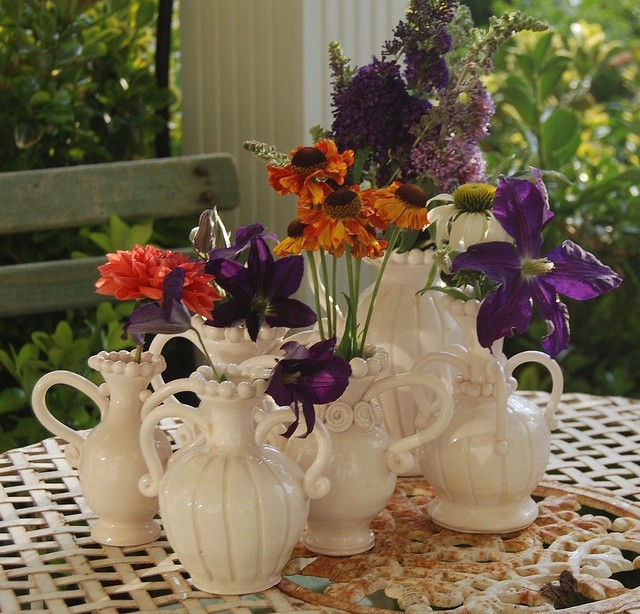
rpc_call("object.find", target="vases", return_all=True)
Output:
[139,363,335,592]
[411,301,565,535]
[308,238,512,478]
[293,342,452,558]
[30,351,207,548]
[148,311,308,422]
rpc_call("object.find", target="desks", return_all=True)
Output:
[0,382,640,613]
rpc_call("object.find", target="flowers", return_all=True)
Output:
[249,334,352,442]
[94,246,227,385]
[394,168,627,373]
[259,143,429,376]
[243,2,550,256]
[195,202,318,346]
